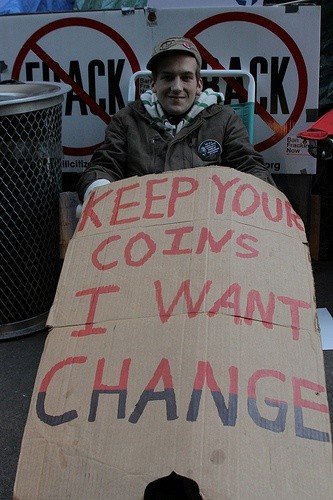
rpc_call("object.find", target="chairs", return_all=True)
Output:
[128,69,254,150]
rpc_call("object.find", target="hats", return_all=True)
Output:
[146,36,202,71]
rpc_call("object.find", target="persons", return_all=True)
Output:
[76,36,278,206]
